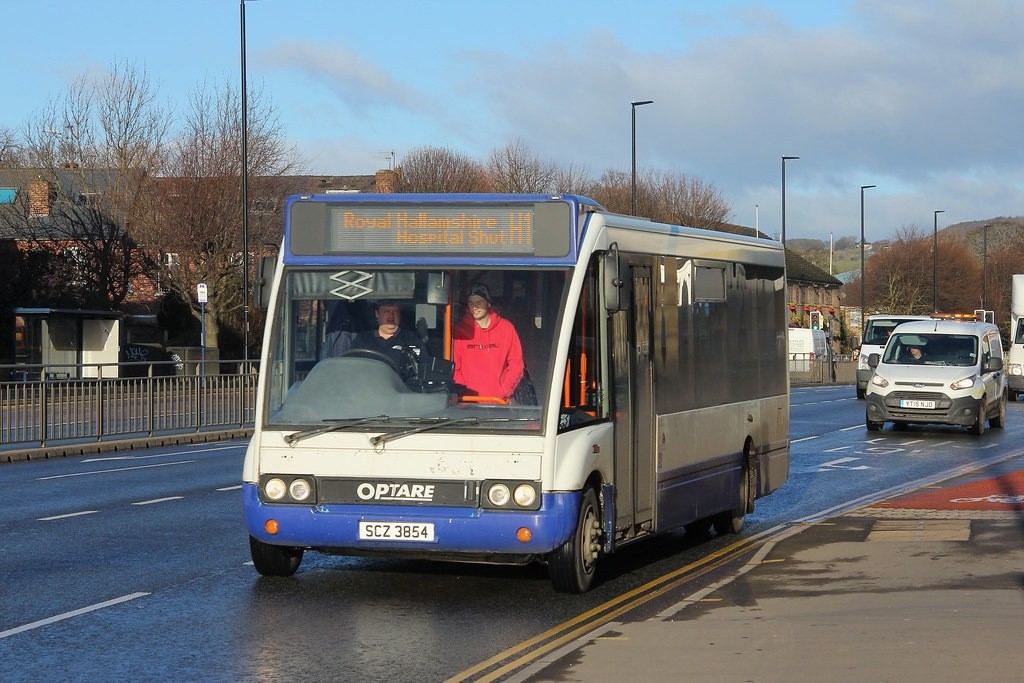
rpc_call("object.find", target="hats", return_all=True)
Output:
[466,282,493,305]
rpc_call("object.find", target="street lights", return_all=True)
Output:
[984,223,992,310]
[934,210,945,314]
[781,156,800,248]
[861,185,876,345]
[631,101,653,217]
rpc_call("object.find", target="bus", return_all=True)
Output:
[242,192,790,592]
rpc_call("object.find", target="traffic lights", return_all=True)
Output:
[810,312,819,330]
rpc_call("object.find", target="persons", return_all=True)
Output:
[900,345,929,365]
[349,299,432,379]
[887,328,894,337]
[452,284,524,405]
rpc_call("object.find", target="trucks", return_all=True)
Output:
[1008,274,1024,402]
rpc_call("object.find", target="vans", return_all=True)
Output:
[866,314,1008,435]
[856,315,941,399]
